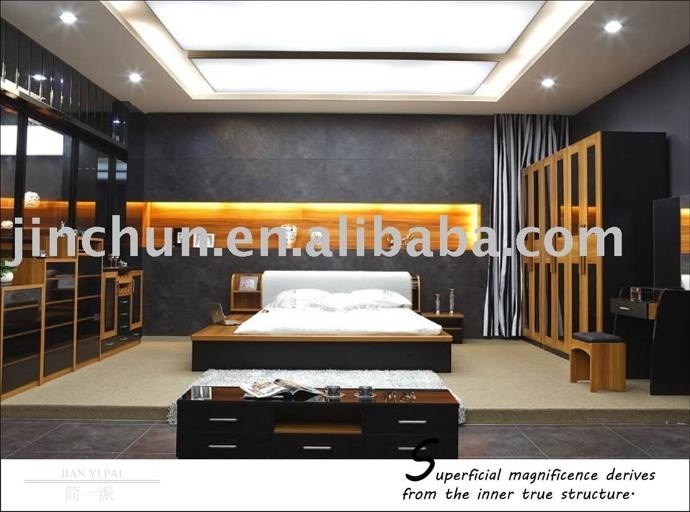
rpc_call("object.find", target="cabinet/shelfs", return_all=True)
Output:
[100,271,118,340]
[130,270,143,332]
[521,131,668,357]
[0,236,102,401]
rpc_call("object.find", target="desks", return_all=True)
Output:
[609,285,690,395]
[173,368,459,458]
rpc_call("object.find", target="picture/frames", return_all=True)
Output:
[238,276,258,291]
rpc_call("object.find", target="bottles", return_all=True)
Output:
[107,255,120,269]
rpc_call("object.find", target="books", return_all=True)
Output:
[240,377,325,402]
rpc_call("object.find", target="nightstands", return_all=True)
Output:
[421,310,464,344]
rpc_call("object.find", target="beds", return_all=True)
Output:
[190,272,454,373]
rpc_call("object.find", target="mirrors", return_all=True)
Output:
[679,208,690,291]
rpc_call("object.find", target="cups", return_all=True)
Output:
[384,389,417,405]
[325,385,372,397]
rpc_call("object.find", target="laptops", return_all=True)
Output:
[208,303,242,326]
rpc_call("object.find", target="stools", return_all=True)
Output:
[567,331,628,392]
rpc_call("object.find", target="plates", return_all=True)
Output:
[322,392,379,400]
